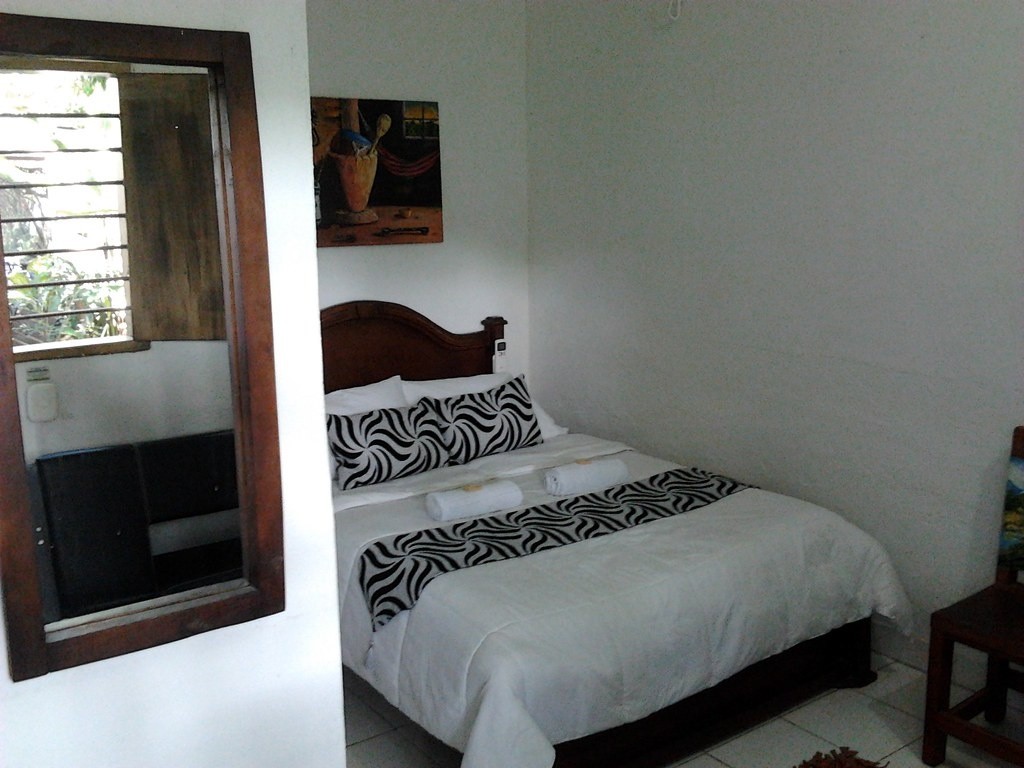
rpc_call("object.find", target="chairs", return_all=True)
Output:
[921,426,1024,768]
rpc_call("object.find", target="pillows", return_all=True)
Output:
[433,372,544,467]
[323,396,449,492]
[401,372,513,407]
[325,375,410,480]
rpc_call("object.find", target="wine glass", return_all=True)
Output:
[328,152,378,225]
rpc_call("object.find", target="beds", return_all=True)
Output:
[319,300,910,768]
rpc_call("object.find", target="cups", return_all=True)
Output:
[398,208,412,218]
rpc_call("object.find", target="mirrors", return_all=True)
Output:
[0,12,286,682]
